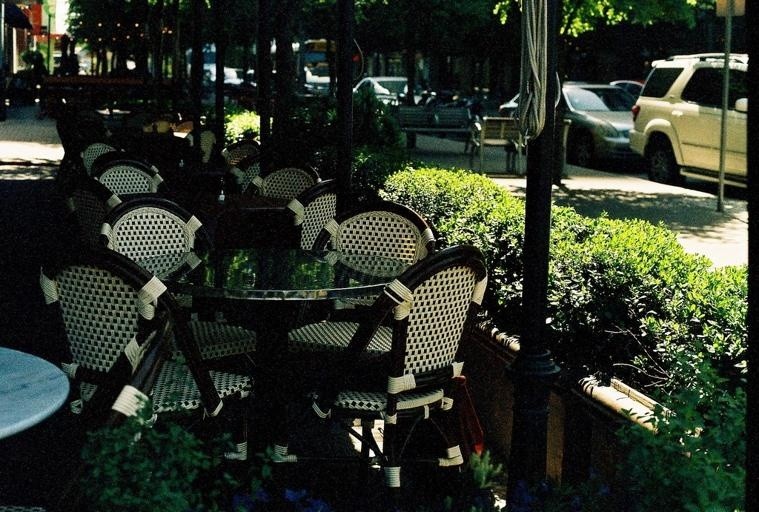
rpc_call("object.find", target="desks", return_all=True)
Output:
[132,246,418,311]
[0,344,69,440]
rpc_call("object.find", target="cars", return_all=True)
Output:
[354,76,424,107]
[496,50,748,197]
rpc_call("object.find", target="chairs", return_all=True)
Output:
[285,198,438,381]
[43,109,345,244]
[269,242,490,512]
[40,242,257,492]
[99,196,259,373]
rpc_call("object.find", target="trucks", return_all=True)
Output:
[271,38,339,96]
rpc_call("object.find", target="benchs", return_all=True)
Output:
[392,104,482,153]
[469,116,572,175]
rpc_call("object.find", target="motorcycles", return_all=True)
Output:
[389,80,490,137]
